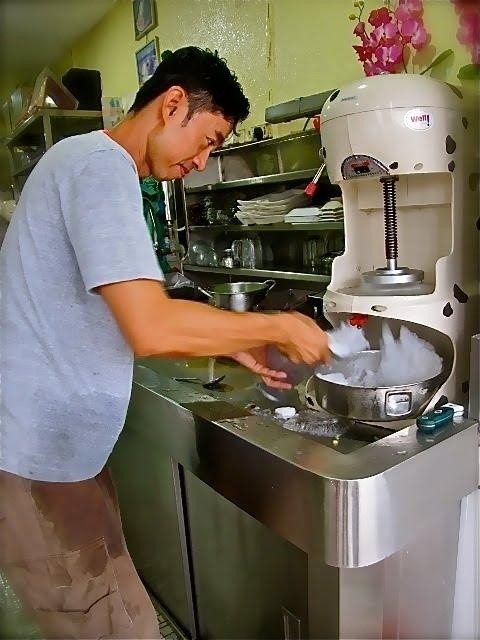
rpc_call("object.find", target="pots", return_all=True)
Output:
[215,279,277,312]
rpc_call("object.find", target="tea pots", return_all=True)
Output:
[230,232,263,268]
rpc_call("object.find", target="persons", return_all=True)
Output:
[1,45,331,640]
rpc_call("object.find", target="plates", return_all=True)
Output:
[234,188,344,223]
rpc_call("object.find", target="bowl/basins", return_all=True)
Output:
[187,206,238,226]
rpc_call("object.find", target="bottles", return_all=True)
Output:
[303,235,320,268]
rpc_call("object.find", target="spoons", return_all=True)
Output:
[169,374,228,390]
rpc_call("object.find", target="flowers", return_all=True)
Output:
[450,1,479,93]
[347,0,462,100]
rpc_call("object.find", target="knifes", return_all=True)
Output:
[416,399,470,430]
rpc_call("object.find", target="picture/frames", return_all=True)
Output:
[134,36,160,89]
[133,0,157,40]
